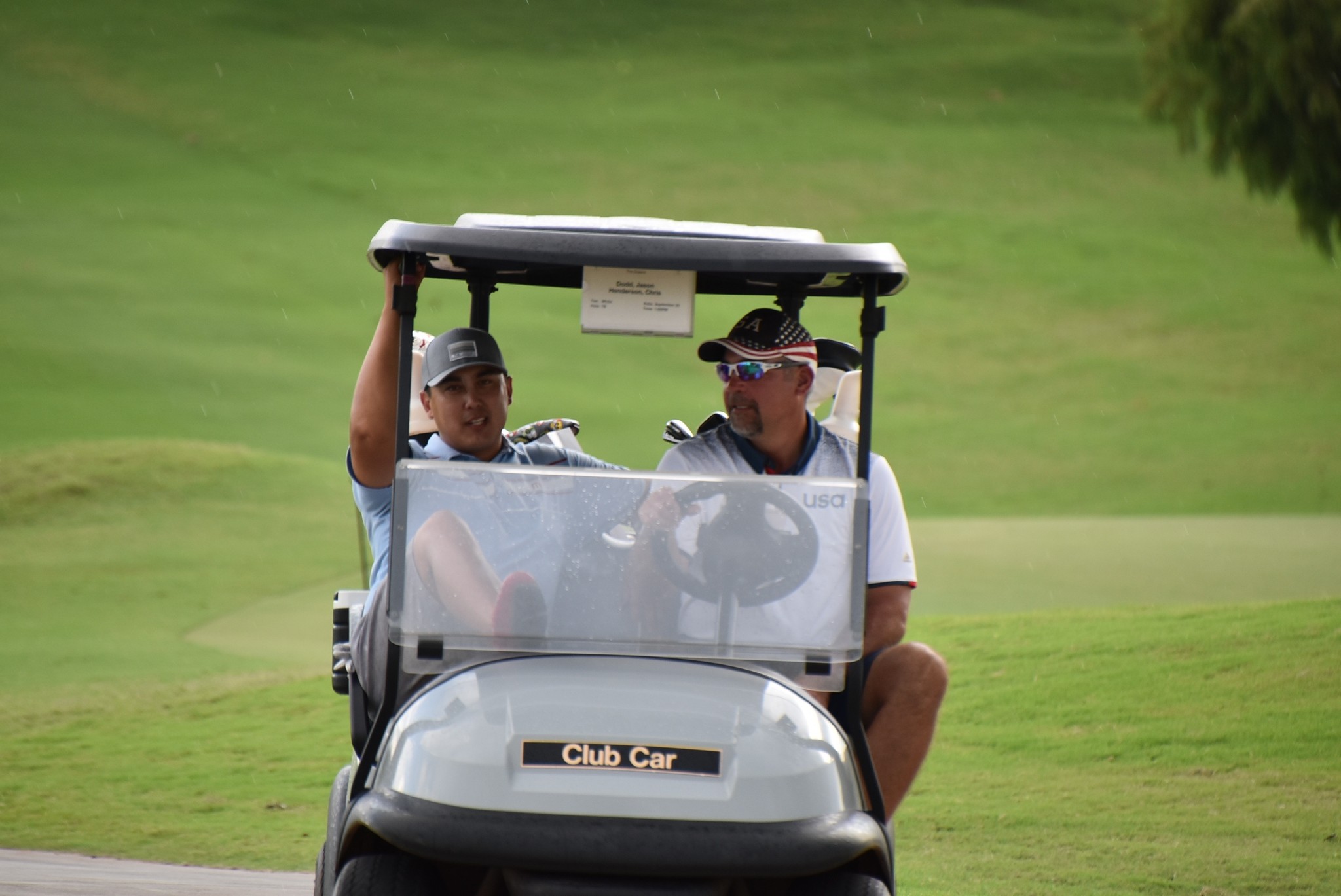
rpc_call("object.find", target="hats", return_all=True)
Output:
[698,309,817,375]
[421,328,507,391]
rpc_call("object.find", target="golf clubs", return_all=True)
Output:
[662,367,862,446]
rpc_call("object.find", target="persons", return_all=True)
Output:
[347,247,649,723]
[628,307,947,823]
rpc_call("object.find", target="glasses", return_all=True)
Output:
[716,361,809,381]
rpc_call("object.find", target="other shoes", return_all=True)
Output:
[495,571,547,636]
